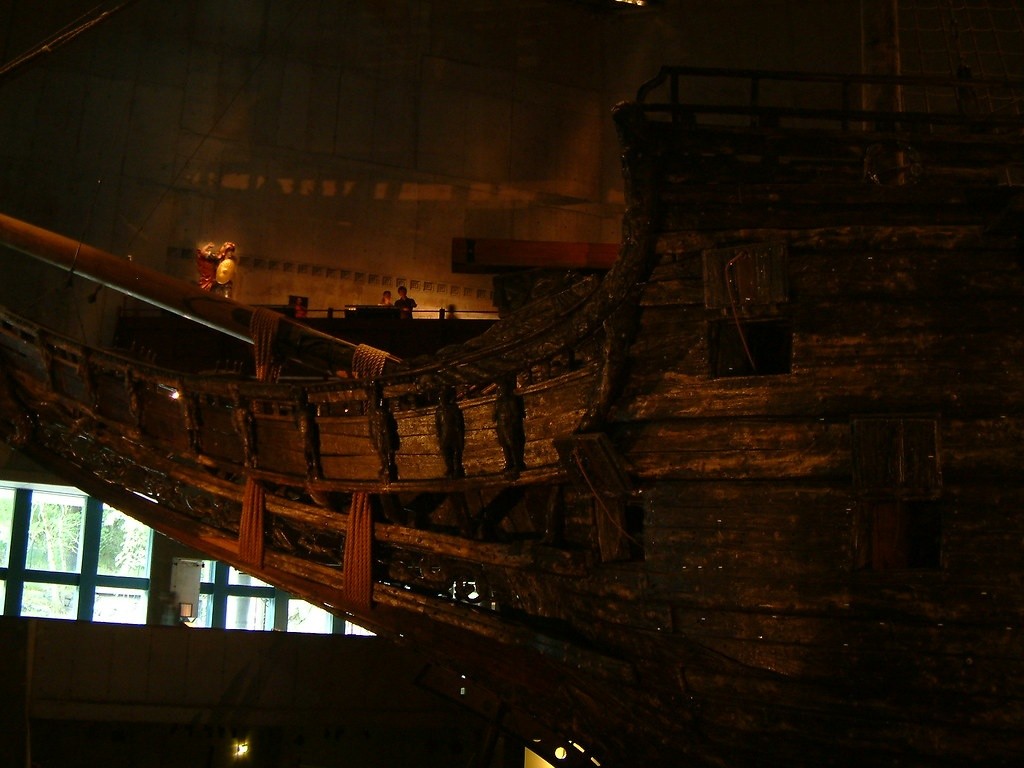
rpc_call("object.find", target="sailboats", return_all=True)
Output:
[1,1,1024,768]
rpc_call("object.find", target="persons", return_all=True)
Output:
[35,331,55,393]
[80,354,102,415]
[364,394,400,483]
[434,390,465,477]
[127,372,144,434]
[199,242,236,299]
[181,389,202,453]
[295,393,320,483]
[446,305,458,320]
[491,377,527,473]
[230,388,259,467]
[381,286,416,320]
